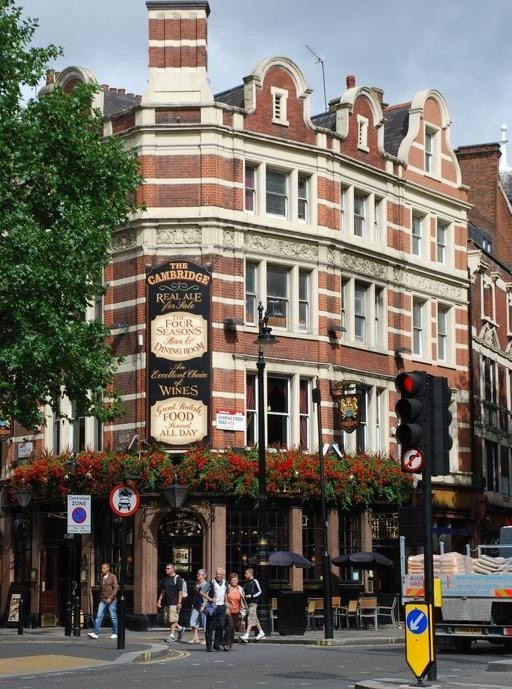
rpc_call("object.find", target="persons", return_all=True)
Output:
[239,567,265,644]
[157,563,186,643]
[88,562,119,639]
[199,568,230,652]
[223,573,248,651]
[186,568,209,645]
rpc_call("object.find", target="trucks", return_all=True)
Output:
[401,527,512,653]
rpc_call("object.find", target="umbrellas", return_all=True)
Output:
[330,551,395,594]
[249,550,313,589]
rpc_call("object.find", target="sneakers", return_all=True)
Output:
[239,636,249,643]
[254,633,265,641]
[206,644,232,652]
[186,638,206,645]
[88,632,98,639]
[109,634,117,639]
[164,635,176,642]
[177,626,185,640]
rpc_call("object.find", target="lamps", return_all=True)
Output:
[159,471,193,511]
[11,479,34,507]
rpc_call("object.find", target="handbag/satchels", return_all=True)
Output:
[202,603,216,616]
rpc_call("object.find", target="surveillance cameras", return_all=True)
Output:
[332,444,343,458]
[128,434,140,450]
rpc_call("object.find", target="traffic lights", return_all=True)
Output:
[428,375,454,452]
[393,370,430,448]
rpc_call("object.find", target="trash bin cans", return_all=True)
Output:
[276,590,307,636]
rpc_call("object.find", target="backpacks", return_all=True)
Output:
[173,574,188,598]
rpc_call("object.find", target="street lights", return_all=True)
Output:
[63,459,82,638]
[255,300,280,636]
[11,479,36,636]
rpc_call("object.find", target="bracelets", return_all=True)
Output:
[251,593,253,599]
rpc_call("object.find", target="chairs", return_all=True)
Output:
[271,584,402,635]
